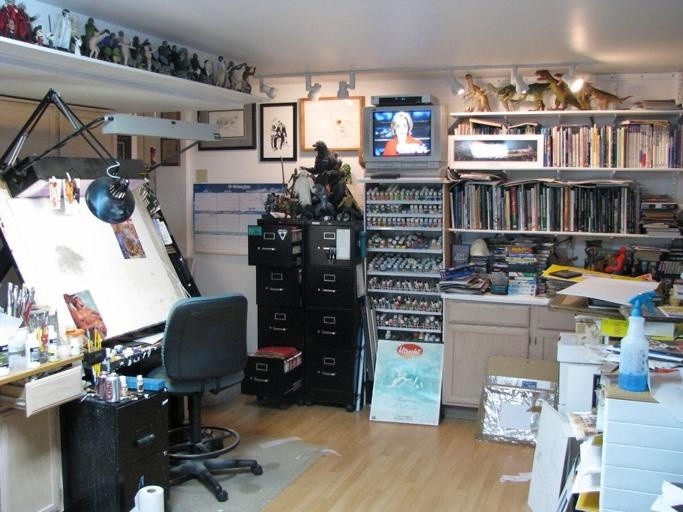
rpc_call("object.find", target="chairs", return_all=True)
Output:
[142,292,262,504]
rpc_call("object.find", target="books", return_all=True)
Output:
[437,238,577,297]
[456,119,681,168]
[583,238,682,376]
[441,166,682,237]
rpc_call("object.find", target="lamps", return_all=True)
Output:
[259,71,359,101]
[0,88,135,225]
[447,64,582,95]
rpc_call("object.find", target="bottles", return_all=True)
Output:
[0,325,84,369]
[366,183,443,342]
[119,375,128,398]
[137,374,143,392]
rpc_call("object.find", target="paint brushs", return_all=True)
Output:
[86,326,101,376]
[7,282,35,328]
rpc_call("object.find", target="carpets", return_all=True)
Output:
[166,431,327,512]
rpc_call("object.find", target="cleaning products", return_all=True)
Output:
[618,290,656,393]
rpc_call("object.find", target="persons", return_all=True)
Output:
[382,111,424,155]
[64,292,105,337]
[64,179,80,215]
[0,0,256,92]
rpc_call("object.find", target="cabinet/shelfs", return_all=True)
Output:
[60,388,171,512]
[0,361,84,512]
[240,218,363,412]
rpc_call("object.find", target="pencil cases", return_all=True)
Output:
[127,376,166,391]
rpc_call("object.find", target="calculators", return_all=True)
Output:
[655,255,683,278]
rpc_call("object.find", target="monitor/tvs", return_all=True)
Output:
[363,104,448,177]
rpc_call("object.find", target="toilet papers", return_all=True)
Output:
[335,229,350,261]
[131,485,163,511]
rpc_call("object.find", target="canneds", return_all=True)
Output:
[96,371,120,403]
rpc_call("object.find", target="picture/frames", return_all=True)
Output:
[195,96,366,161]
